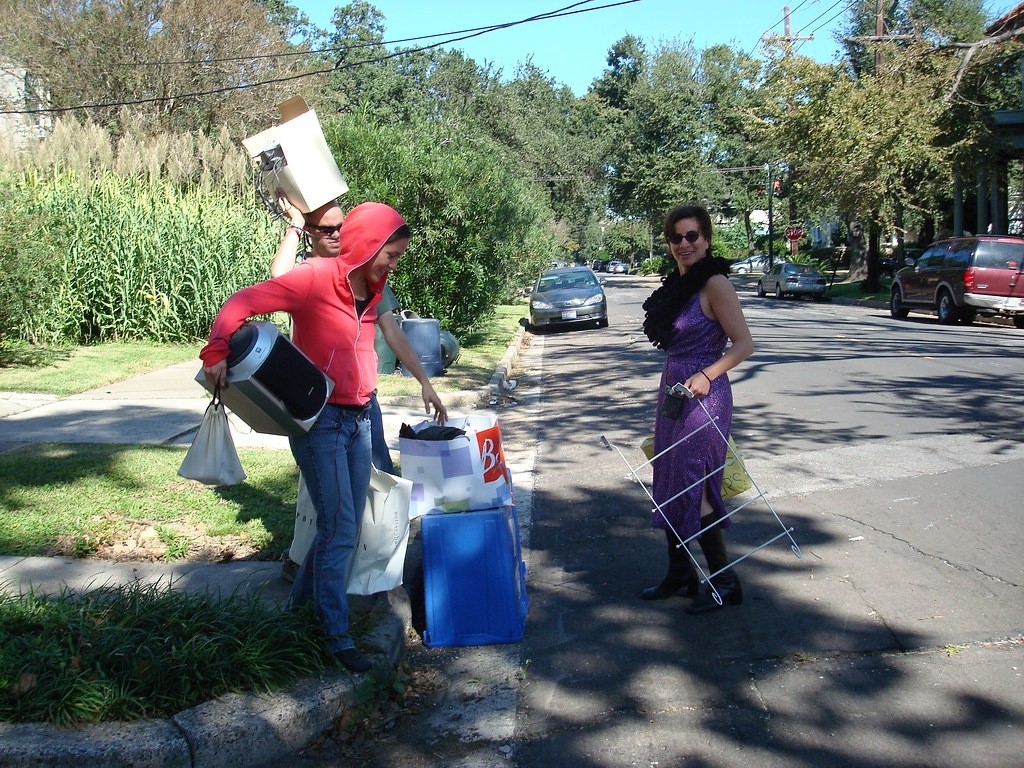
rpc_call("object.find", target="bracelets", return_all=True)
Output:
[700,370,712,384]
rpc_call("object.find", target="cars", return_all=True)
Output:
[589,258,630,275]
[730,255,786,274]
[523,266,610,331]
[757,262,827,301]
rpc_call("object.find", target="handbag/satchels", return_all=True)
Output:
[177,379,248,486]
[399,414,516,521]
[288,461,414,595]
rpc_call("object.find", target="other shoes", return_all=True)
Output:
[333,648,373,672]
[280,559,300,583]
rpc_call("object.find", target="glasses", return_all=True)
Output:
[667,230,703,245]
[305,222,342,235]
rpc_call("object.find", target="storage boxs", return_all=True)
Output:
[422,496,530,647]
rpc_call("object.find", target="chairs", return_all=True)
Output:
[575,278,584,283]
[544,279,556,288]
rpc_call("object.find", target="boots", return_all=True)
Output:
[684,512,743,614]
[640,524,701,601]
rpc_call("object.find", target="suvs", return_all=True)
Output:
[889,234,1024,330]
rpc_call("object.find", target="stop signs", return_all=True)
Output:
[785,225,804,241]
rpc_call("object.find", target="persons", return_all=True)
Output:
[640,205,755,614]
[199,202,410,673]
[270,196,449,584]
